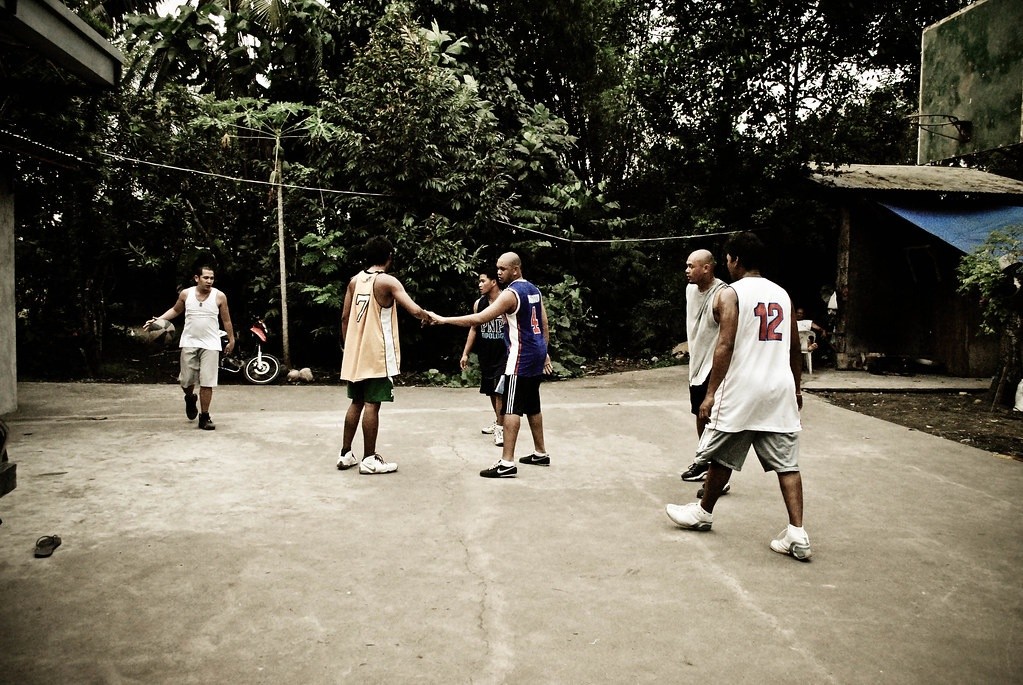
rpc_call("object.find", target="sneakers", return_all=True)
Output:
[336,448,358,469]
[519,451,551,466]
[482,421,502,434]
[695,481,731,498]
[359,451,399,474]
[492,426,504,446]
[184,393,198,421]
[479,460,518,478]
[769,528,812,560]
[681,462,710,481]
[198,412,216,430]
[665,502,713,532]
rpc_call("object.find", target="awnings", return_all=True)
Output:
[879,198,1023,275]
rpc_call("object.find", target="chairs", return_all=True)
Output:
[798,331,816,375]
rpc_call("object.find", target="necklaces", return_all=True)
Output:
[195,288,210,307]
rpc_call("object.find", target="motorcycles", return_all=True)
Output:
[218,319,281,385]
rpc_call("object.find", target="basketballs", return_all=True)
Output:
[148,319,176,345]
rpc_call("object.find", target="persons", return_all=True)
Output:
[420,251,553,478]
[796,308,827,352]
[336,234,431,475]
[459,269,510,447]
[681,249,732,499]
[664,230,813,561]
[143,265,235,430]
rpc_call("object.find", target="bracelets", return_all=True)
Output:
[796,393,802,397]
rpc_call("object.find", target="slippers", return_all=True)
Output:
[34,533,61,558]
